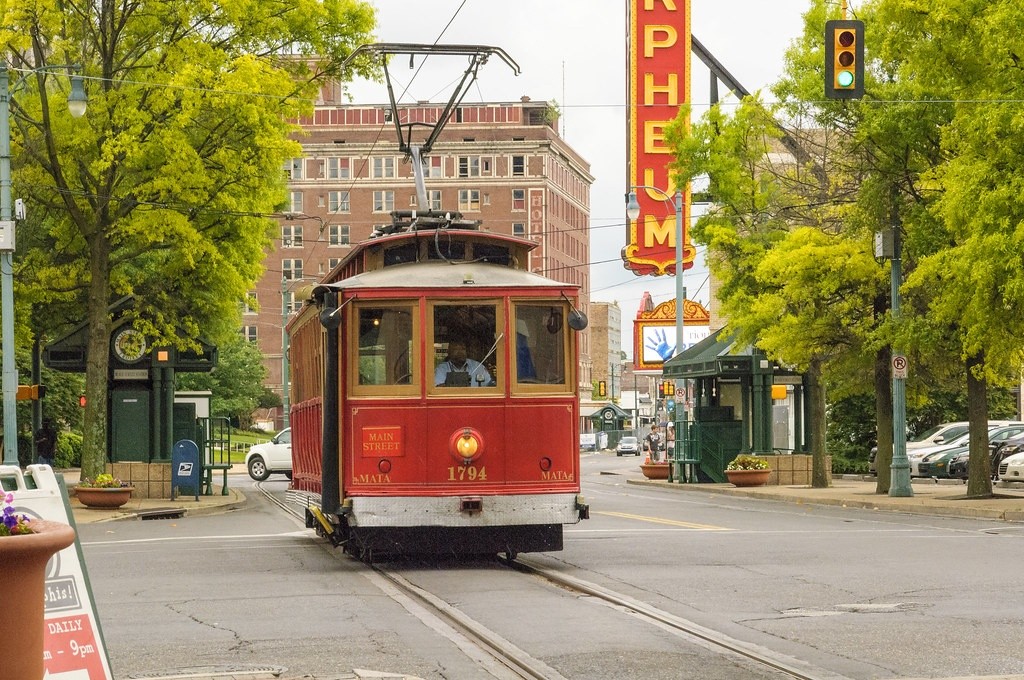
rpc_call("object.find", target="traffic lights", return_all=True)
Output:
[834,28,856,90]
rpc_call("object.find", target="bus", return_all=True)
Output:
[284,208,591,570]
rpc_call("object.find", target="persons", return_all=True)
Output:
[646,425,663,460]
[434,341,497,387]
[664,422,675,459]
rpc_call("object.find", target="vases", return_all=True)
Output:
[639,465,673,479]
[724,469,771,487]
[73,486,134,509]
[0,520,75,680]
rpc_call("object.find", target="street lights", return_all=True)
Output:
[0,57,88,466]
[626,185,689,480]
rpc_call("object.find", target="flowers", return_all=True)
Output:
[645,461,668,465]
[79,474,128,488]
[727,454,769,471]
[0,491,36,536]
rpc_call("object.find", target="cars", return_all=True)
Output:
[643,435,666,451]
[616,437,640,457]
[866,419,1024,482]
[245,426,296,481]
